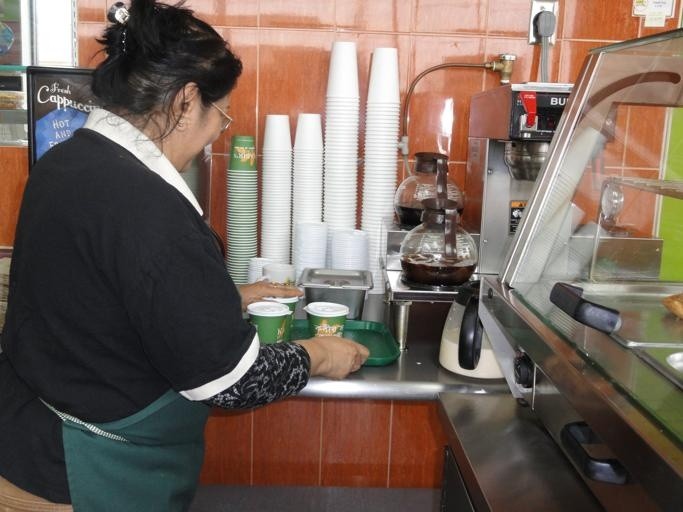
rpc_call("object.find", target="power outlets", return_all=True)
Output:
[529,0,560,47]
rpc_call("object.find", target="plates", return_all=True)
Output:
[289,318,402,368]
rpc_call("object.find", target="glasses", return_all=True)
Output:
[210,101,232,133]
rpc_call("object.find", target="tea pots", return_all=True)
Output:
[437,279,531,380]
[394,152,465,227]
[398,195,480,289]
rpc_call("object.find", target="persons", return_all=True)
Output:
[0,0,371,512]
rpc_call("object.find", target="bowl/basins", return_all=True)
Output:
[331,229,370,272]
[293,222,328,284]
[298,268,375,322]
[247,258,285,284]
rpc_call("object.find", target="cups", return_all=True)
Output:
[292,111,323,221]
[324,40,360,267]
[246,301,293,344]
[256,263,295,286]
[361,47,401,295]
[223,136,259,285]
[303,301,350,339]
[259,114,292,263]
[263,295,300,328]
